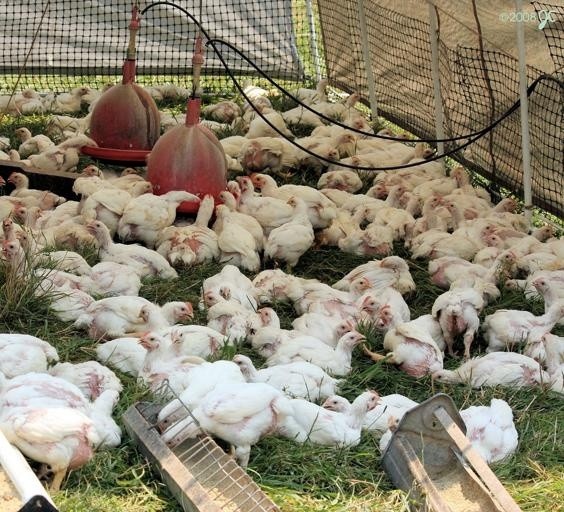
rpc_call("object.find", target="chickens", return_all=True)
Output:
[0,79,564,494]
